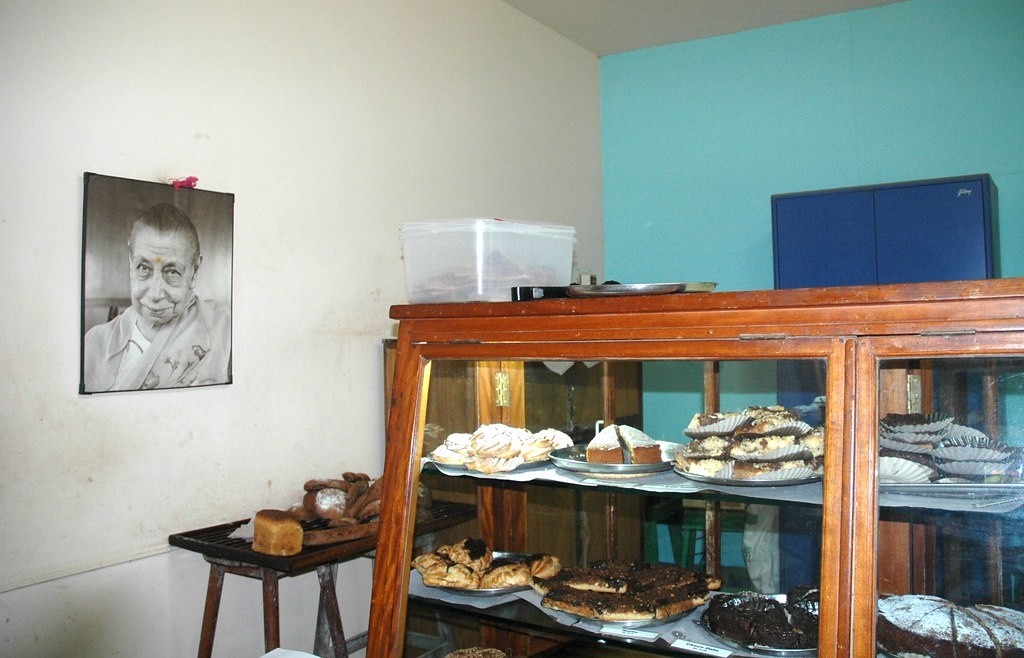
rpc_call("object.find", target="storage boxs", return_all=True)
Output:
[398,219,577,303]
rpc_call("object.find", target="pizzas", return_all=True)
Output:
[531,559,723,621]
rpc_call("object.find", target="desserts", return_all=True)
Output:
[675,405,824,481]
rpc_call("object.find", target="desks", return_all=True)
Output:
[167,497,475,658]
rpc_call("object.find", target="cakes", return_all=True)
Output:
[709,584,820,650]
[872,593,1024,658]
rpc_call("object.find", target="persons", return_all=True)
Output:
[85,203,232,393]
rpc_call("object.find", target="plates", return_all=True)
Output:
[565,607,692,628]
[674,466,824,485]
[439,551,545,597]
[426,453,550,468]
[565,282,719,297]
[547,440,686,474]
[878,482,1024,498]
[700,607,818,657]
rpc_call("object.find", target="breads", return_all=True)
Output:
[430,422,575,474]
[251,472,432,556]
[586,424,665,464]
[411,537,561,589]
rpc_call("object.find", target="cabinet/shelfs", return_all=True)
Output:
[360,278,1024,658]
[770,170,1002,596]
[378,333,644,658]
